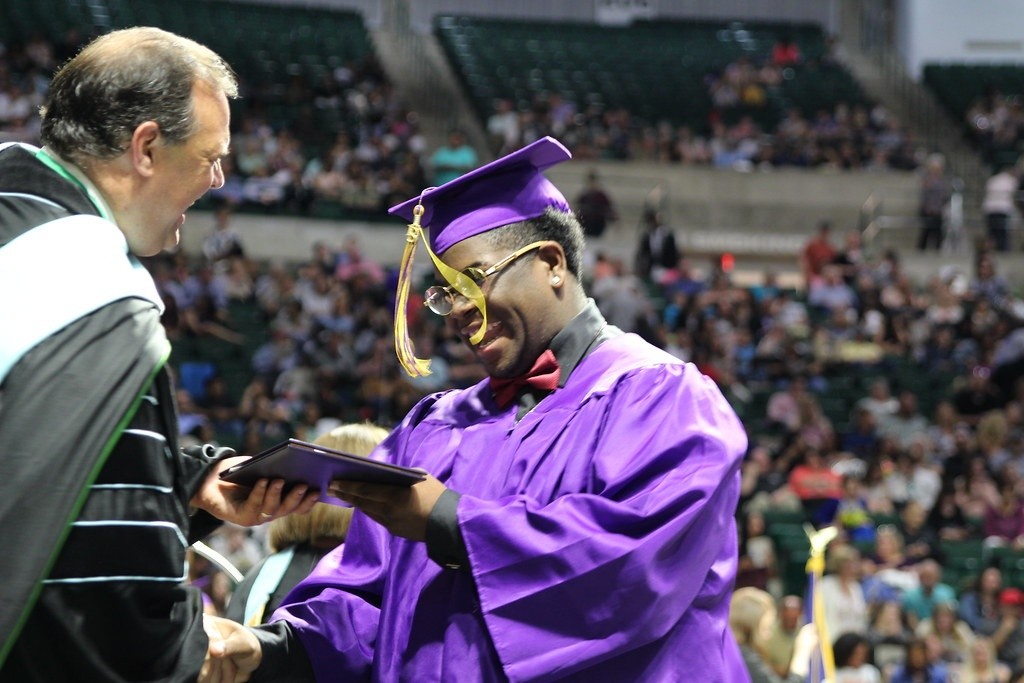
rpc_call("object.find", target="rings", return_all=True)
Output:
[260,512,274,519]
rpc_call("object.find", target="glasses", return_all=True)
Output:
[419,241,562,316]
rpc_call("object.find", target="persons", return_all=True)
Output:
[0,28,1024,683]
[0,26,322,683]
[210,137,749,683]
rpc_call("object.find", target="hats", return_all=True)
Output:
[387,137,574,377]
